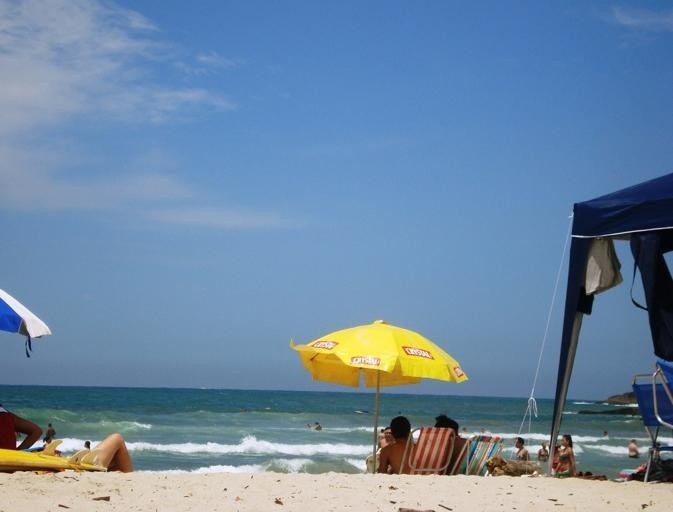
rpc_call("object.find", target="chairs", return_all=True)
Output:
[630,359,673,483]
[399,426,506,475]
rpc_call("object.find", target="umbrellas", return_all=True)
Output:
[287,320,469,473]
[0,288,52,359]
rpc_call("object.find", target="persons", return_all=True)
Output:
[46,423,56,439]
[84,441,90,450]
[82,433,133,474]
[44,437,51,448]
[549,442,562,471]
[514,437,530,461]
[376,413,469,476]
[537,442,548,462]
[0,404,43,449]
[628,439,639,459]
[554,435,576,477]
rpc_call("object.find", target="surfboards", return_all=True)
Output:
[0,439,107,472]
